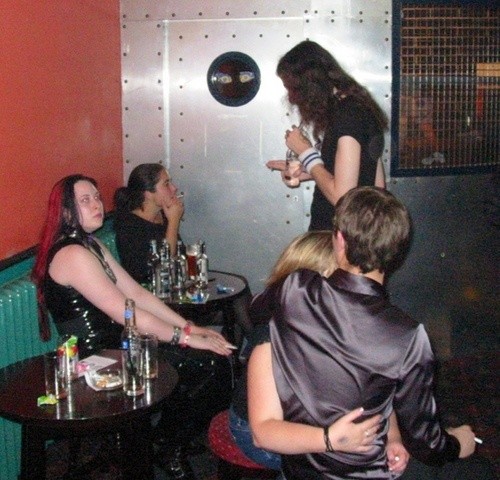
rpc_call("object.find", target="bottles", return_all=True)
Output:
[147,238,209,299]
[283,148,301,188]
[120,298,140,391]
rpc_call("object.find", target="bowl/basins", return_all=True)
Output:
[93,369,122,388]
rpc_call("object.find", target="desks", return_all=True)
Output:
[167,271,249,389]
[0,349,173,480]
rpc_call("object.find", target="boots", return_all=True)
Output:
[202,318,246,403]
[154,390,228,480]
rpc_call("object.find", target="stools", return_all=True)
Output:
[207,411,277,480]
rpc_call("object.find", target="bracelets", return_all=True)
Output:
[323,424,334,452]
[298,147,324,174]
[181,320,192,348]
[173,326,181,347]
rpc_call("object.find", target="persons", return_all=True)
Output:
[228,185,476,480]
[112,163,253,375]
[31,173,239,480]
[266,42,389,233]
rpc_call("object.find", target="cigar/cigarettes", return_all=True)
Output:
[176,195,184,198]
[474,437,483,444]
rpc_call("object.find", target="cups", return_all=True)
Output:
[57,334,79,382]
[122,341,145,398]
[137,334,159,378]
[44,349,69,399]
